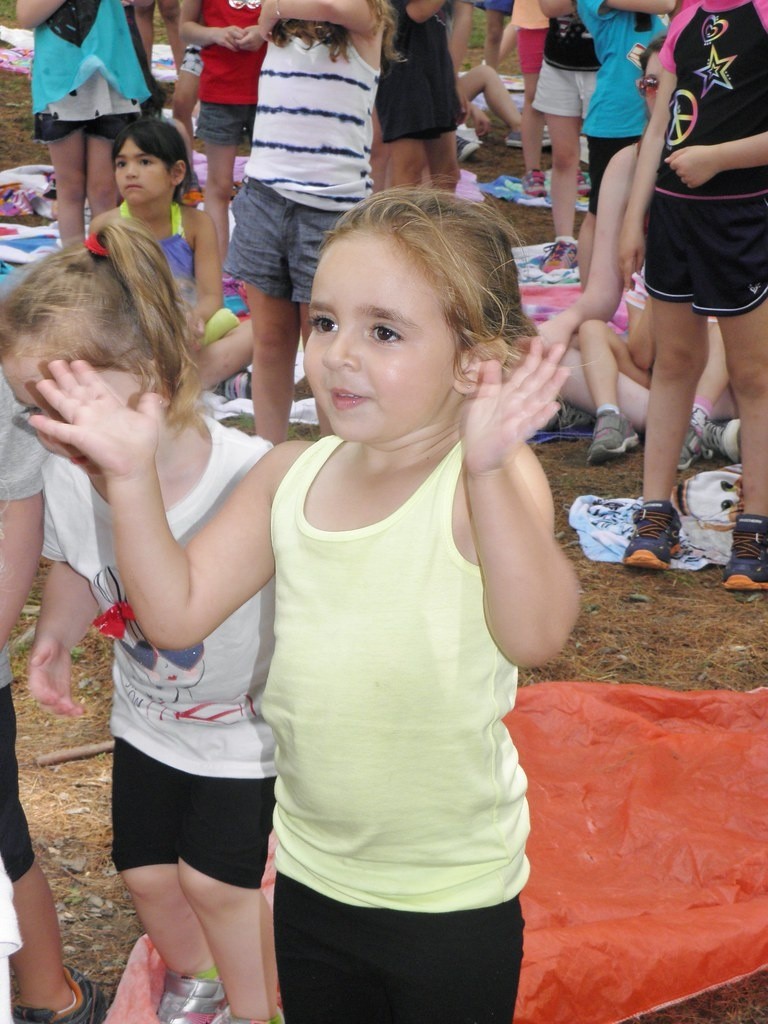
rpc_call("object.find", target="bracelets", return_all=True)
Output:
[276,0,283,19]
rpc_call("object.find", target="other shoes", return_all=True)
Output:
[219,370,253,400]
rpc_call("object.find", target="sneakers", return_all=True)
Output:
[456,135,481,163]
[521,168,548,198]
[504,131,552,148]
[720,512,767,592]
[180,169,205,207]
[12,964,107,1024]
[621,498,682,571]
[697,416,742,463]
[576,166,593,196]
[158,968,225,1024]
[540,238,576,272]
[676,426,702,469]
[587,404,640,465]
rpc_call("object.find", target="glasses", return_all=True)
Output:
[634,74,660,98]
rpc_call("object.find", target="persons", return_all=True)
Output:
[27,171,582,1024]
[0,216,286,1024]
[15,0,768,468]
[617,0,768,593]
[0,348,108,1024]
